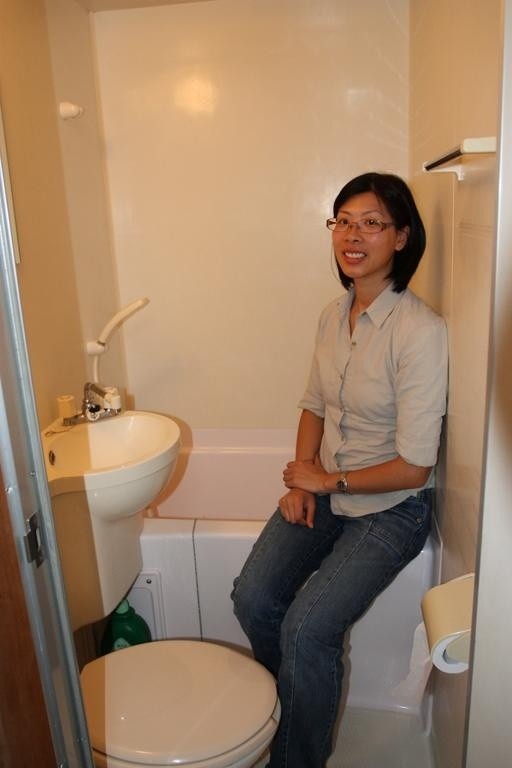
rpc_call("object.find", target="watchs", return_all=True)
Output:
[336,470,352,496]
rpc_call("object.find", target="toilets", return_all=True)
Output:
[80,638,281,768]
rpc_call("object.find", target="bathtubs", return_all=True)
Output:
[136,444,435,715]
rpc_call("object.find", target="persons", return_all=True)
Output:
[228,170,449,768]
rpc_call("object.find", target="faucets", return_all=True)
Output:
[84,381,123,416]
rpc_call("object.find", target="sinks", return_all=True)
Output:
[47,410,181,521]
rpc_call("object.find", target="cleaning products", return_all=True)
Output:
[101,600,151,654]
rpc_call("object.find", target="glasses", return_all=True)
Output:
[326,218,396,234]
